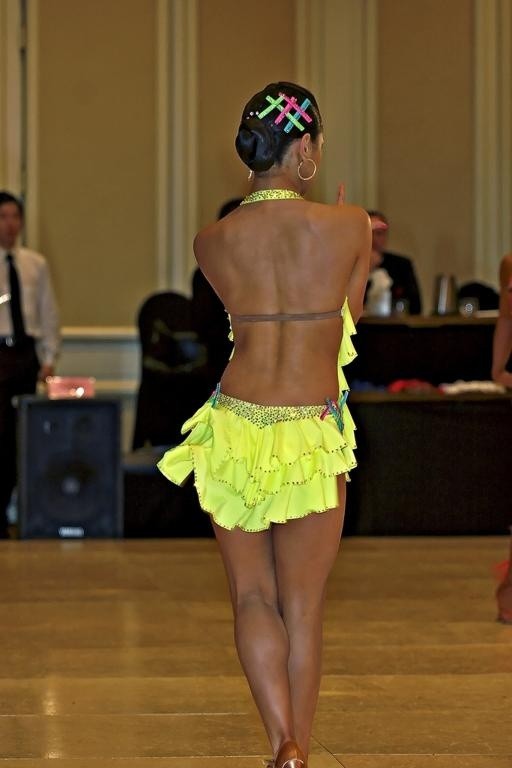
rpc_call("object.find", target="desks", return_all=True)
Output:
[343,304,512,382]
[344,375,512,538]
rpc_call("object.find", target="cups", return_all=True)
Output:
[393,299,408,319]
[456,293,480,320]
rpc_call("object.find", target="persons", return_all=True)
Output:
[366,213,423,317]
[493,254,512,623]
[156,82,373,768]
[190,198,247,298]
[0,189,59,541]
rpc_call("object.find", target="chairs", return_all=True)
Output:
[129,287,206,451]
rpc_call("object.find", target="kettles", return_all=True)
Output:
[431,274,458,319]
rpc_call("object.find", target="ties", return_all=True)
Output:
[6,254,27,347]
